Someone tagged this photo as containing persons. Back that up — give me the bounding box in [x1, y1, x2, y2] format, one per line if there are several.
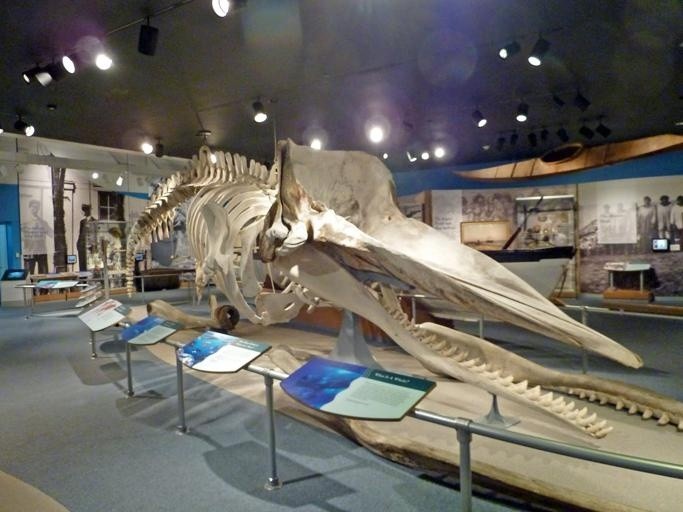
[600, 194, 683, 253]
[76, 202, 96, 273]
[21, 199, 54, 274]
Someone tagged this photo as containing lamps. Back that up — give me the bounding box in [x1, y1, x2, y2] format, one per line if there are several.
[252, 99, 268, 123]
[596, 124, 612, 138]
[509, 133, 519, 147]
[407, 150, 418, 162]
[495, 137, 505, 151]
[154, 142, 166, 159]
[515, 102, 529, 123]
[557, 128, 569, 142]
[498, 42, 521, 60]
[471, 110, 487, 128]
[552, 96, 566, 108]
[541, 129, 548, 140]
[11, 1, 246, 89]
[572, 95, 592, 112]
[527, 39, 553, 68]
[528, 133, 537, 147]
[16, 122, 35, 137]
[579, 126, 595, 139]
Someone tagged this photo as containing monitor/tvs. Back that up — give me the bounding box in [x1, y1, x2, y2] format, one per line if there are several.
[135, 253, 144, 262]
[651, 237, 669, 252]
[2, 268, 28, 281]
[66, 255, 77, 265]
